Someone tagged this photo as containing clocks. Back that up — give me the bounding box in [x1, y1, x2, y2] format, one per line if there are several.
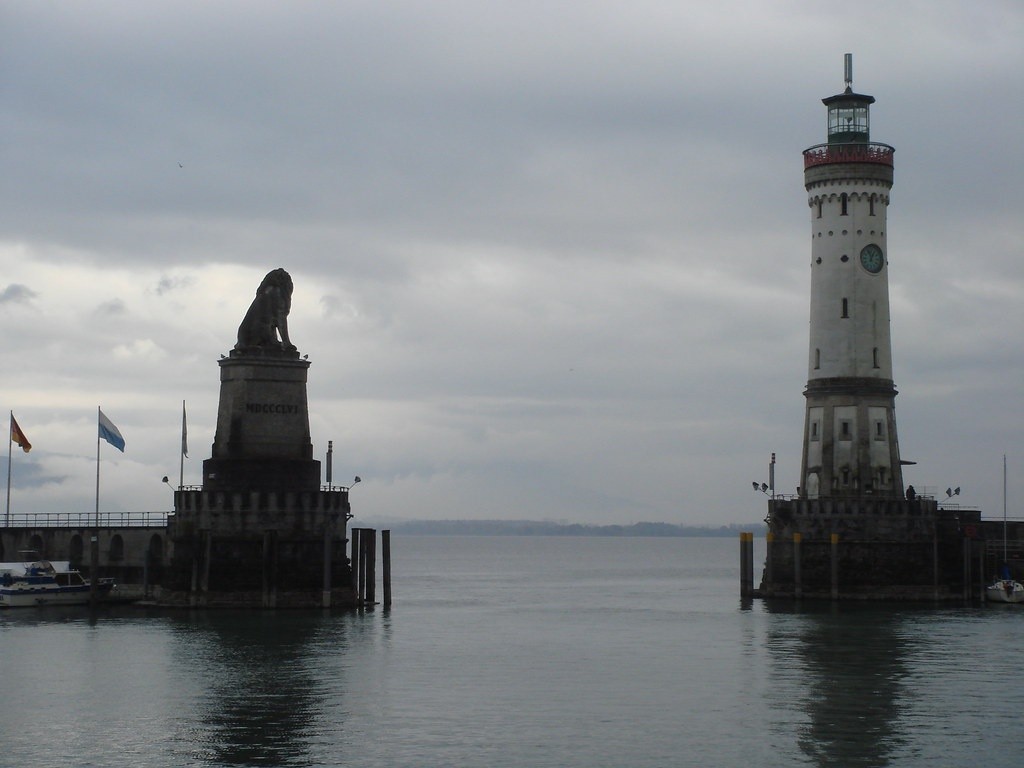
[861, 244, 883, 273]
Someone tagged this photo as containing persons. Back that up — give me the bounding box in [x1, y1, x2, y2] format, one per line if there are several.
[906, 485, 917, 500]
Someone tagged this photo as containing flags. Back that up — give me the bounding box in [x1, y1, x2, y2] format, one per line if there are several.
[182, 405, 189, 459]
[99, 411, 125, 454]
[11, 414, 32, 453]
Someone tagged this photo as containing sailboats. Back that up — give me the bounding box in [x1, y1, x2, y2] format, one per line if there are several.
[985, 453, 1024, 604]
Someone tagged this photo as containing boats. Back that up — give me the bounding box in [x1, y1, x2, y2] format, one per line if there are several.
[0, 559, 120, 607]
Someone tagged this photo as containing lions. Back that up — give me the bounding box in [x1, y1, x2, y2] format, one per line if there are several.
[235, 268, 298, 350]
